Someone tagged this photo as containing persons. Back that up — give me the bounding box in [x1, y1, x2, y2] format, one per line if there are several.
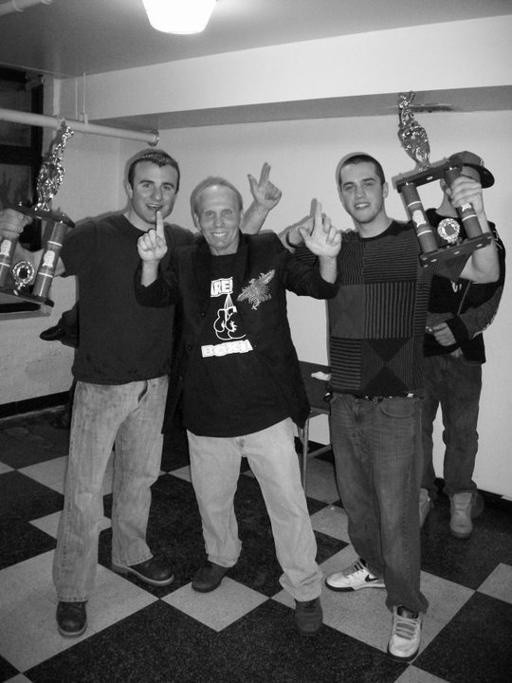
[137, 174, 346, 635]
[272, 149, 503, 662]
[0, 147, 282, 638]
[405, 150, 508, 540]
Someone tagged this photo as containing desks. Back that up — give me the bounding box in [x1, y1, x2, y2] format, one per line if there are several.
[298, 359, 331, 499]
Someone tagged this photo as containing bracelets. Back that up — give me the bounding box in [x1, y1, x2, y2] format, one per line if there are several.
[283, 227, 299, 251]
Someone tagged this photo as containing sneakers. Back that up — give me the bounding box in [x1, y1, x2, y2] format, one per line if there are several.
[420, 488, 433, 530]
[325, 559, 387, 592]
[386, 604, 423, 663]
[448, 491, 478, 540]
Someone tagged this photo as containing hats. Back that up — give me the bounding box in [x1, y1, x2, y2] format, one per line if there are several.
[446, 151, 495, 189]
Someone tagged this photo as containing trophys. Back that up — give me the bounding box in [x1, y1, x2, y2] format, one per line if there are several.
[389, 89, 496, 272]
[1, 115, 80, 308]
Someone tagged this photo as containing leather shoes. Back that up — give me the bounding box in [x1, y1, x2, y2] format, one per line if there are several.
[56, 599, 88, 637]
[109, 557, 175, 587]
[292, 597, 323, 638]
[192, 557, 229, 593]
